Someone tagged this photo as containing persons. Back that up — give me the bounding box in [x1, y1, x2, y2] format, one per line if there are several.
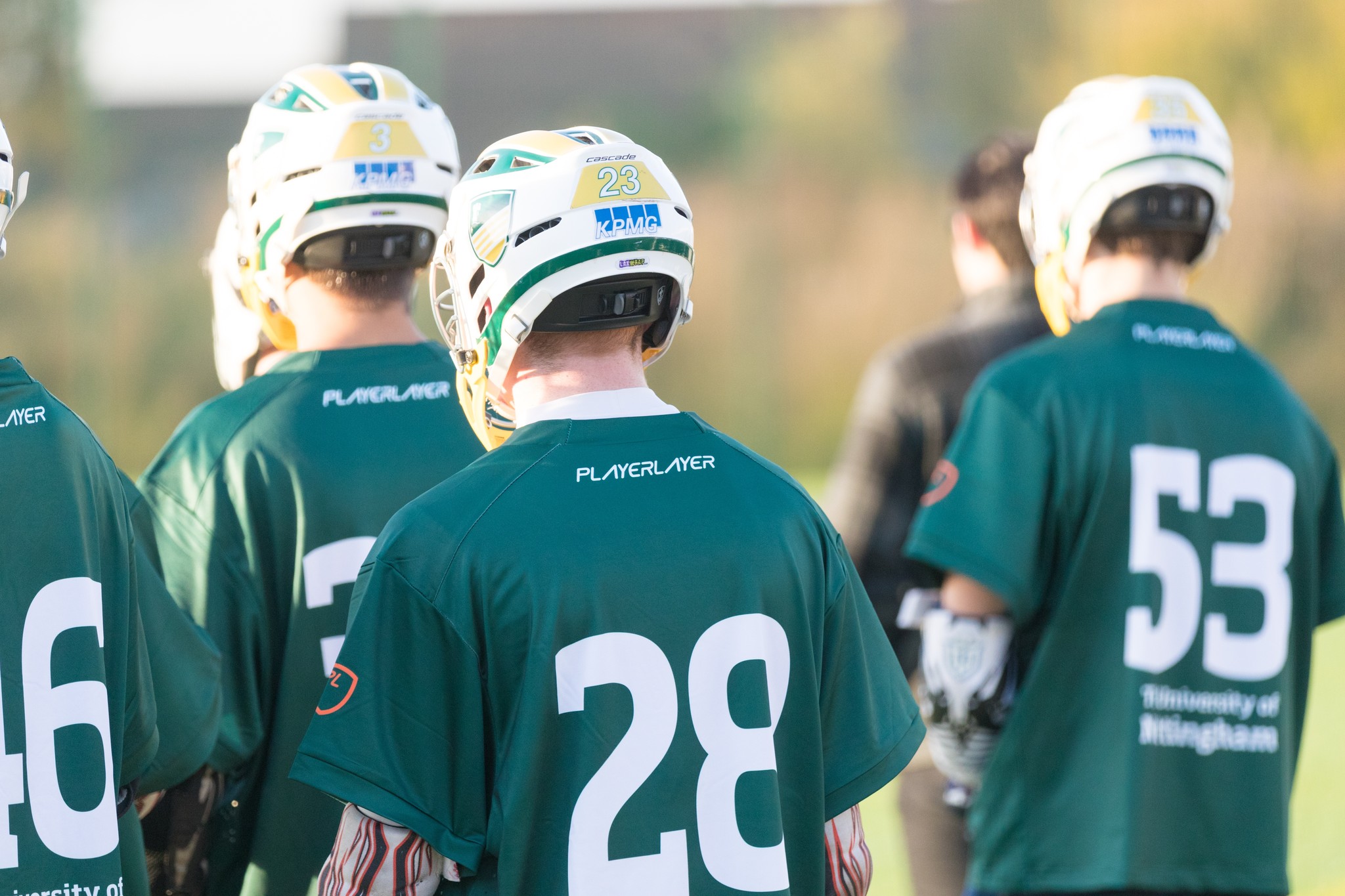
[818, 134, 1053, 896]
[905, 76, 1345, 896]
[0, 64, 927, 896]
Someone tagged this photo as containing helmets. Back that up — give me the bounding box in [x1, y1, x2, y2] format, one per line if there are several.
[1019, 73, 1236, 339]
[228, 61, 459, 349]
[429, 124, 696, 451]
[0, 119, 29, 259]
[210, 211, 272, 390]
